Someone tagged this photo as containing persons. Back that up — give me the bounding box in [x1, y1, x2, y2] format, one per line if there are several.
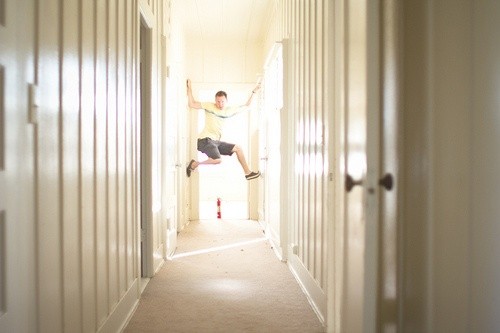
[183, 79, 262, 180]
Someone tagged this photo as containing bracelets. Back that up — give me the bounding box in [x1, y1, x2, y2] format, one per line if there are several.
[252, 90, 256, 93]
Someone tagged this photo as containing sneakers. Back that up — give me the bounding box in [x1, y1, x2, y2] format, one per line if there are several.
[185, 160, 195, 178]
[246, 172, 261, 180]
